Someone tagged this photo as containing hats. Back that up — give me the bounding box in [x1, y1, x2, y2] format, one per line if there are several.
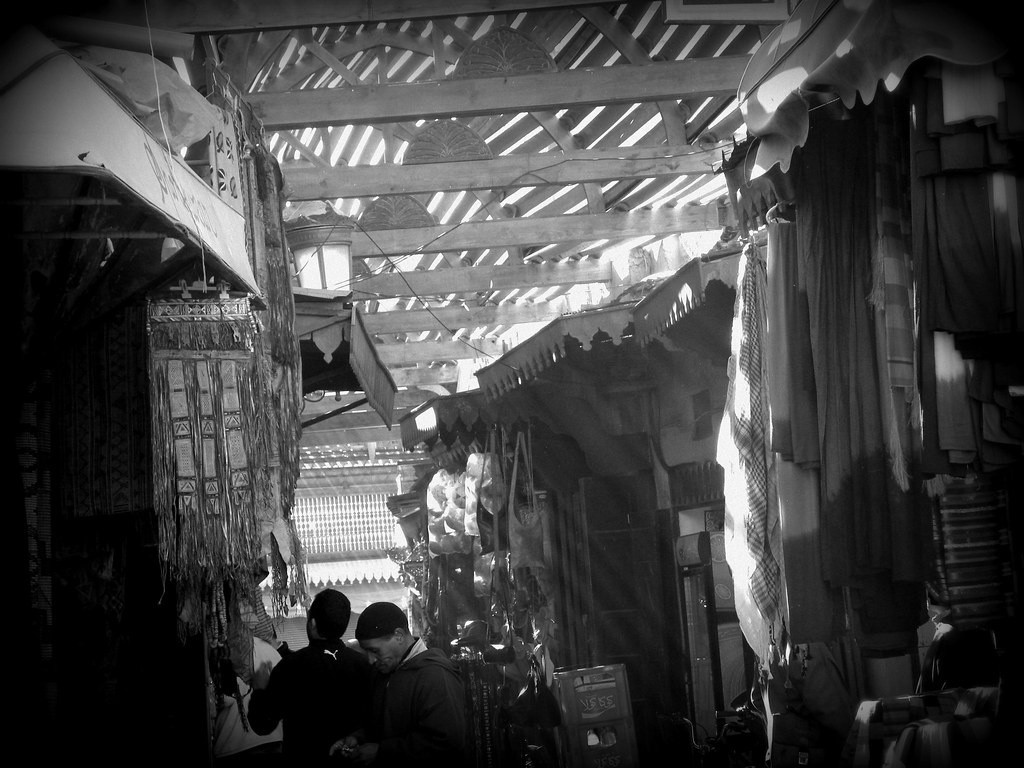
[356, 603, 408, 642]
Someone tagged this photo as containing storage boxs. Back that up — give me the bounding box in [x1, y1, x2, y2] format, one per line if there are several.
[553, 659, 638, 768]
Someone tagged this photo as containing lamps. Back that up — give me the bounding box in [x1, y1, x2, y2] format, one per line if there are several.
[286, 202, 357, 304]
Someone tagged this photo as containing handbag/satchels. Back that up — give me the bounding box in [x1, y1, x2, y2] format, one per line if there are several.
[478, 422, 559, 569]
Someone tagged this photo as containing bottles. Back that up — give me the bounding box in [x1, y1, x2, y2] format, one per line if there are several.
[587, 728, 617, 747]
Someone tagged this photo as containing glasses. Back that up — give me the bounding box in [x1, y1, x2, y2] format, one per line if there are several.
[307, 611, 312, 620]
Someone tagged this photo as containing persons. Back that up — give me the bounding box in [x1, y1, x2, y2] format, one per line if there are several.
[329, 602, 467, 768]
[211, 588, 376, 768]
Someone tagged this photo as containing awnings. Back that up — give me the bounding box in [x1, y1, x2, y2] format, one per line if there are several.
[740, 0, 1024, 181]
[294, 288, 398, 430]
[472, 301, 635, 403]
[1, 29, 265, 310]
[258, 557, 400, 592]
[632, 240, 764, 348]
[400, 390, 484, 449]
